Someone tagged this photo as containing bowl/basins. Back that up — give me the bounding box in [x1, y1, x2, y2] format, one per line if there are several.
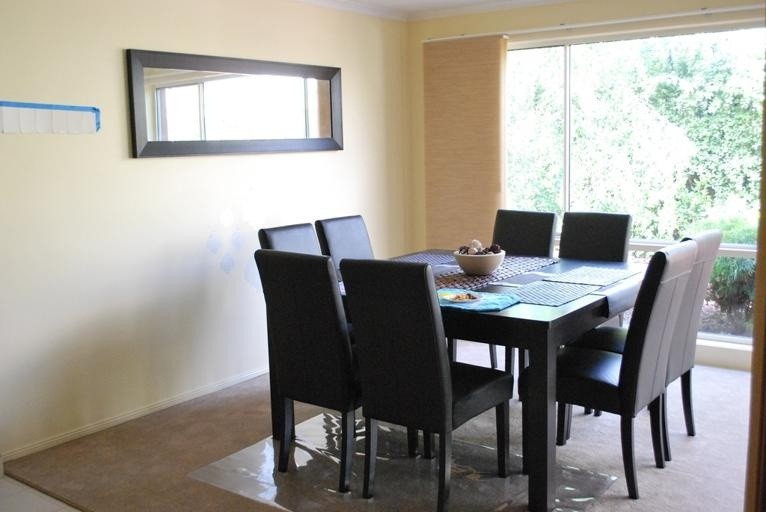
[453, 249, 506, 275]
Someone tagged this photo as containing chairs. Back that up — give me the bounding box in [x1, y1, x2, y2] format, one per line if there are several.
[314, 215, 374, 283]
[339, 258, 513, 511]
[517, 240, 697, 499]
[254, 249, 418, 493]
[258, 222, 322, 256]
[584, 232, 721, 461]
[447, 209, 555, 373]
[518, 212, 630, 416]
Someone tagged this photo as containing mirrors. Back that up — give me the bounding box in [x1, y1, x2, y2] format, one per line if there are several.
[126, 49, 342, 158]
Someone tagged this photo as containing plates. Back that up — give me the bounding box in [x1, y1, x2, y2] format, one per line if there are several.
[443, 293, 482, 303]
